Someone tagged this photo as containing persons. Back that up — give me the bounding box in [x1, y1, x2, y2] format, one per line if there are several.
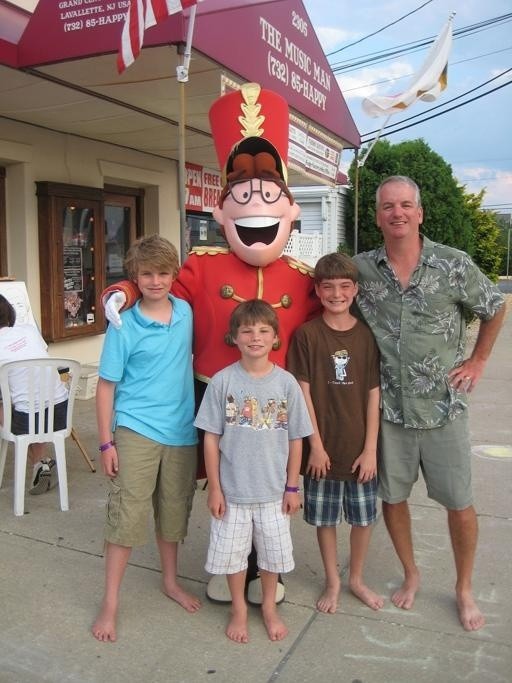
[0, 295, 71, 497]
[92, 237, 200, 641]
[97, 82, 329, 606]
[192, 299, 314, 644]
[337, 176, 505, 631]
[288, 253, 388, 616]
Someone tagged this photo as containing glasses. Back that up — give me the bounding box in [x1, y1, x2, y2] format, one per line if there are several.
[222, 177, 289, 204]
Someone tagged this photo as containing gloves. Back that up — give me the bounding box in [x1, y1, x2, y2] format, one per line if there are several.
[103, 290, 128, 330]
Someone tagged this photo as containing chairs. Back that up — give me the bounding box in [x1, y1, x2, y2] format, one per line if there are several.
[0, 357, 82, 517]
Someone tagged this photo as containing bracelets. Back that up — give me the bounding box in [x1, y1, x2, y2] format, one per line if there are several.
[97, 442, 113, 449]
[284, 486, 298, 492]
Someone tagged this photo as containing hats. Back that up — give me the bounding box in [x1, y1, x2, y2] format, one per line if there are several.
[205, 81, 289, 189]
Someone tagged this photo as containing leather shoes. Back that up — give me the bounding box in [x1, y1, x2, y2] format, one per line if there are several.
[245, 545, 287, 607]
[203, 556, 241, 609]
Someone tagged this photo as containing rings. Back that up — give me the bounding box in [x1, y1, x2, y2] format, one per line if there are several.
[464, 377, 471, 382]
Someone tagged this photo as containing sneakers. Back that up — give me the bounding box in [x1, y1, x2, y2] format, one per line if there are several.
[27, 459, 51, 496]
[48, 456, 59, 491]
